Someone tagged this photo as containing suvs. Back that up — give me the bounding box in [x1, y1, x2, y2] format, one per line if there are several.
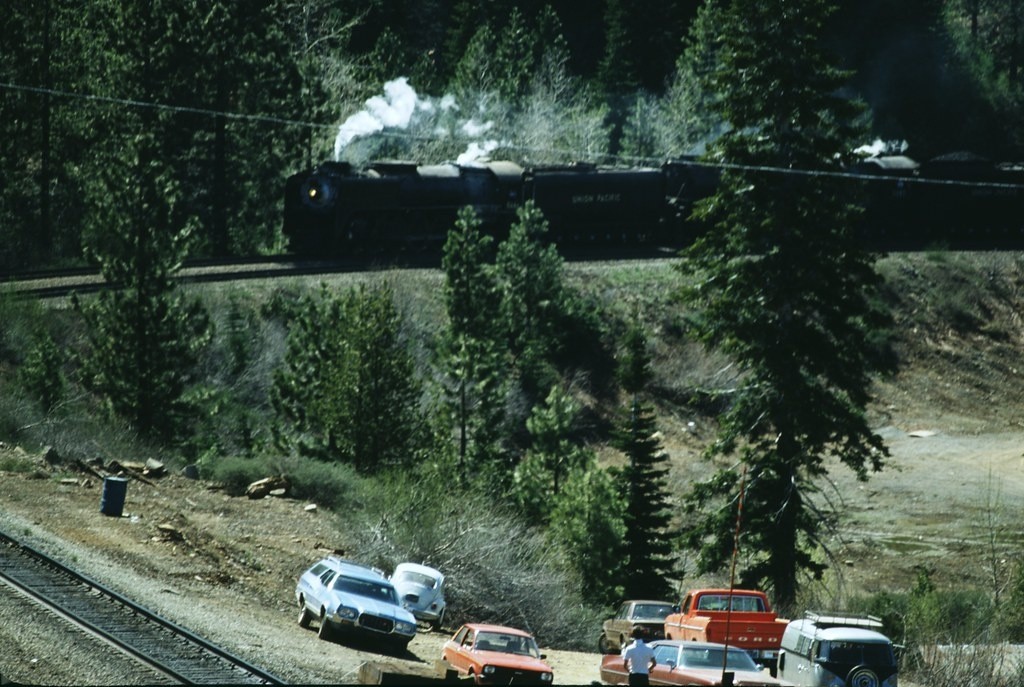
[294, 556, 416, 653]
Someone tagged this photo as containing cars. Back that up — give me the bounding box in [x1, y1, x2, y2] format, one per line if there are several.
[441, 625, 555, 686]
[601, 641, 793, 687]
[597, 600, 680, 655]
[382, 563, 448, 631]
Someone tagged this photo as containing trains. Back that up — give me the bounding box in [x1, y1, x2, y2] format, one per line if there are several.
[280, 153, 1024, 267]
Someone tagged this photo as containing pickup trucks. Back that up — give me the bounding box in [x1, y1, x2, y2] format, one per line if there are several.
[664, 590, 791, 673]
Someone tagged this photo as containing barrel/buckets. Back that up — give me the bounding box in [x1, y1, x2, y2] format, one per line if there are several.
[101, 477, 128, 517]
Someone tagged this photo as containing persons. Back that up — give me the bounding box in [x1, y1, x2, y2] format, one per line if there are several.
[623, 627, 656, 687]
